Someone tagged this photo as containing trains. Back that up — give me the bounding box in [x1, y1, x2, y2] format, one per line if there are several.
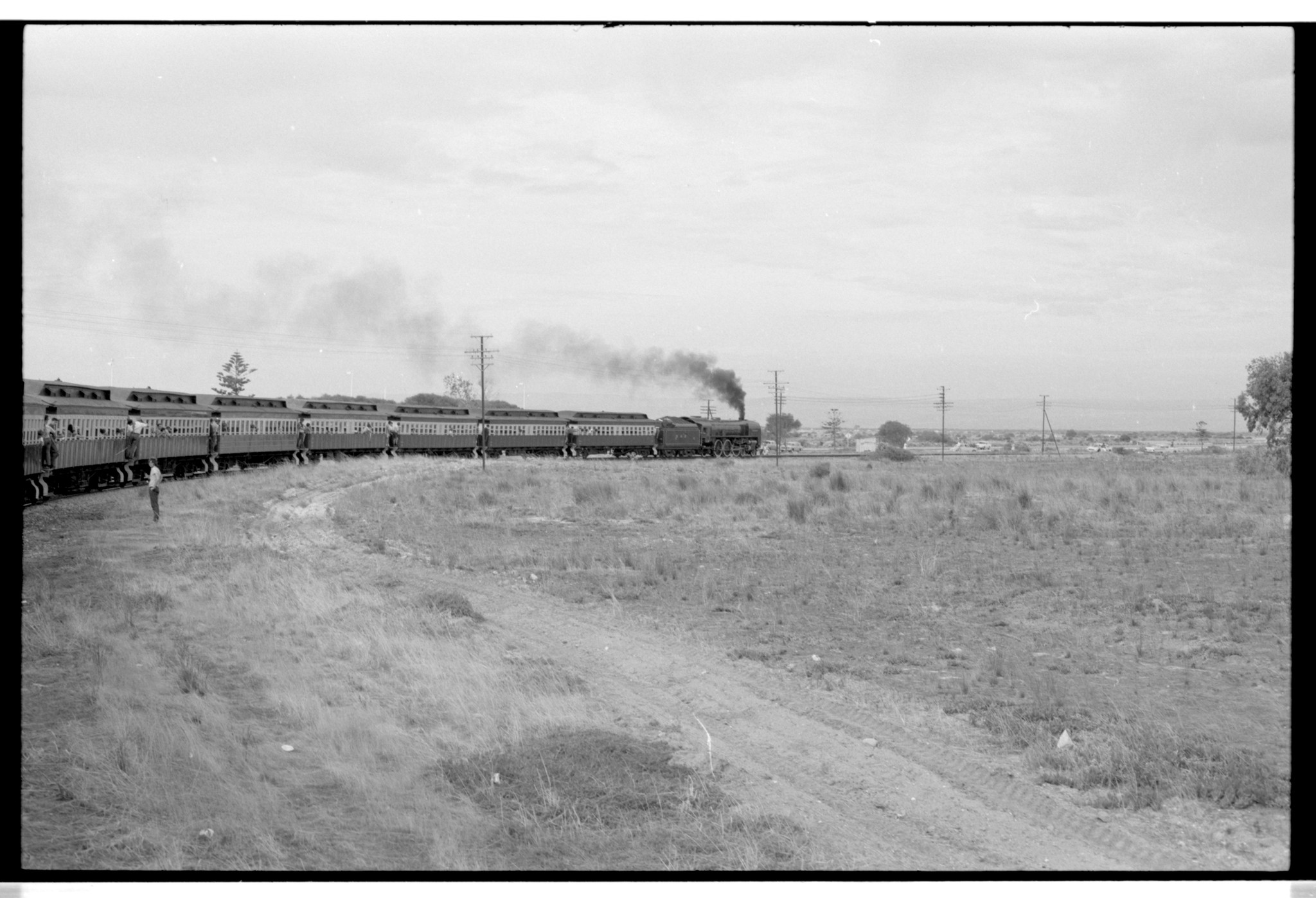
[22, 392, 761, 503]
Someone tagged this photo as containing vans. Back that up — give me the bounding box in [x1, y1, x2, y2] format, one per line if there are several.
[760, 440, 778, 451]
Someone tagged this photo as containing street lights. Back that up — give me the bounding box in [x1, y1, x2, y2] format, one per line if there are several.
[347, 371, 353, 396]
[519, 382, 525, 411]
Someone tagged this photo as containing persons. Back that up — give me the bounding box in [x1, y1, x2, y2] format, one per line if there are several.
[151, 423, 177, 438]
[297, 418, 310, 448]
[144, 457, 163, 523]
[481, 424, 489, 444]
[126, 417, 149, 460]
[580, 428, 598, 435]
[385, 420, 401, 447]
[449, 428, 457, 437]
[564, 423, 577, 447]
[759, 443, 769, 454]
[38, 415, 59, 469]
[62, 424, 124, 439]
[362, 424, 372, 434]
[411, 428, 415, 435]
[209, 418, 220, 456]
[222, 423, 227, 431]
[250, 425, 258, 433]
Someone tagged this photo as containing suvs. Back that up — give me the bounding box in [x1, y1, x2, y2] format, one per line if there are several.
[952, 443, 974, 453]
[779, 442, 803, 452]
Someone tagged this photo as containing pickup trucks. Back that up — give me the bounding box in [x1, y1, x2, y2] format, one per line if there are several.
[1145, 444, 1179, 454]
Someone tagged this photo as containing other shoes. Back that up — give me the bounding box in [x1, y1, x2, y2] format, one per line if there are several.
[126, 459, 138, 466]
[41, 464, 54, 473]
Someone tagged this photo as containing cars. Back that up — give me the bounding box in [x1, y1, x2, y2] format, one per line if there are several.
[1086, 443, 1111, 453]
[976, 441, 991, 450]
